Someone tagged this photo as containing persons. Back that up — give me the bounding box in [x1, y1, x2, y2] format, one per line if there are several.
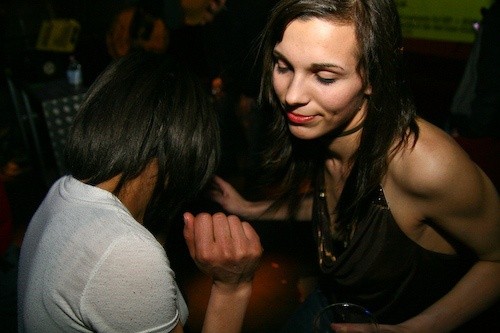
[208, 0, 500, 333]
[16, 48, 263, 333]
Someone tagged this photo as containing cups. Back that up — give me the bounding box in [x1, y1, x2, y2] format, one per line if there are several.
[313, 302, 377, 333]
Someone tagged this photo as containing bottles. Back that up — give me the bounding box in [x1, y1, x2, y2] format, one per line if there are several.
[64, 57, 82, 90]
